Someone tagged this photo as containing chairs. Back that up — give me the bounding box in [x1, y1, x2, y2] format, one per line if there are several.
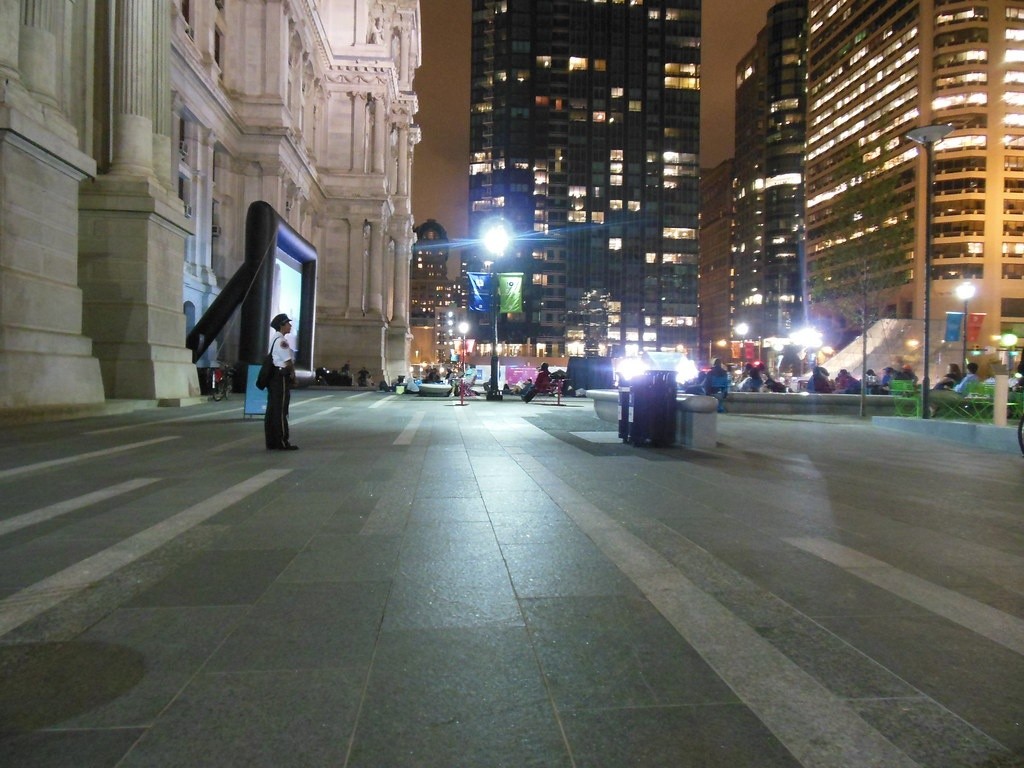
[889, 380, 920, 418]
[966, 383, 993, 424]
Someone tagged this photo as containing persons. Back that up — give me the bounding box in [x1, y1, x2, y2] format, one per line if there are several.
[700, 359, 983, 413]
[256, 314, 299, 451]
[316, 361, 535, 398]
[521, 362, 551, 403]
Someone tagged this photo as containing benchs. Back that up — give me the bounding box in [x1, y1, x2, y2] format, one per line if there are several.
[585, 388, 720, 448]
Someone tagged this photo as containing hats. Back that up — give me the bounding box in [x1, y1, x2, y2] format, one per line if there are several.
[883, 367, 893, 372]
[902, 364, 911, 370]
[271, 313, 292, 330]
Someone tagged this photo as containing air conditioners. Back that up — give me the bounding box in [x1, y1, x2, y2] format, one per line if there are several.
[184, 205, 193, 217]
[217, 0, 226, 10]
[211, 225, 222, 236]
[179, 140, 190, 156]
[286, 201, 292, 211]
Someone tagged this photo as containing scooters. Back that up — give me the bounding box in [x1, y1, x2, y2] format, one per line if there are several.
[707, 388, 727, 413]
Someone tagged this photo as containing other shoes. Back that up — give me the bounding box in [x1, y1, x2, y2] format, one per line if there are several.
[277, 445, 297, 451]
[521, 394, 527, 402]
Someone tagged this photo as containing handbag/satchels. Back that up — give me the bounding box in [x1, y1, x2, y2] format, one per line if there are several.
[256, 337, 282, 390]
[550, 369, 568, 379]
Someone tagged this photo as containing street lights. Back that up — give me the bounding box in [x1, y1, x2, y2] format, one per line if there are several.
[1000, 336, 1018, 379]
[956, 281, 975, 381]
[478, 223, 510, 401]
[737, 322, 749, 373]
[457, 321, 470, 405]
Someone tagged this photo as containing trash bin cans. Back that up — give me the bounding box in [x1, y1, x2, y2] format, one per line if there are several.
[616, 368, 678, 447]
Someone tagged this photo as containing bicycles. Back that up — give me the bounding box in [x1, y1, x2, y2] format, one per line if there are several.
[210, 363, 237, 402]
